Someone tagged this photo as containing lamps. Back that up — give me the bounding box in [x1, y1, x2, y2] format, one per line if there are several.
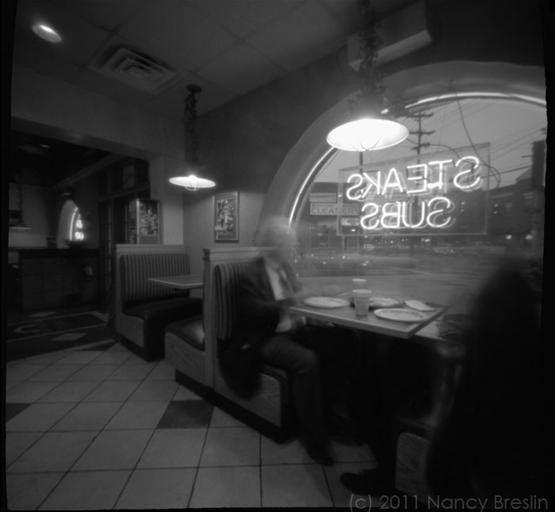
[165, 82, 217, 191]
[8, 174, 32, 230]
[325, 0, 410, 154]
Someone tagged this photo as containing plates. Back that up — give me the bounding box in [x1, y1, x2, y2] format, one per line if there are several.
[303, 293, 426, 325]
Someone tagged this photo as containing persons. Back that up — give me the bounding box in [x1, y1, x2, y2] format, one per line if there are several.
[237, 215, 338, 466]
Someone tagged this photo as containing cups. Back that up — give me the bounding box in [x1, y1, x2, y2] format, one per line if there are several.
[350, 277, 373, 318]
